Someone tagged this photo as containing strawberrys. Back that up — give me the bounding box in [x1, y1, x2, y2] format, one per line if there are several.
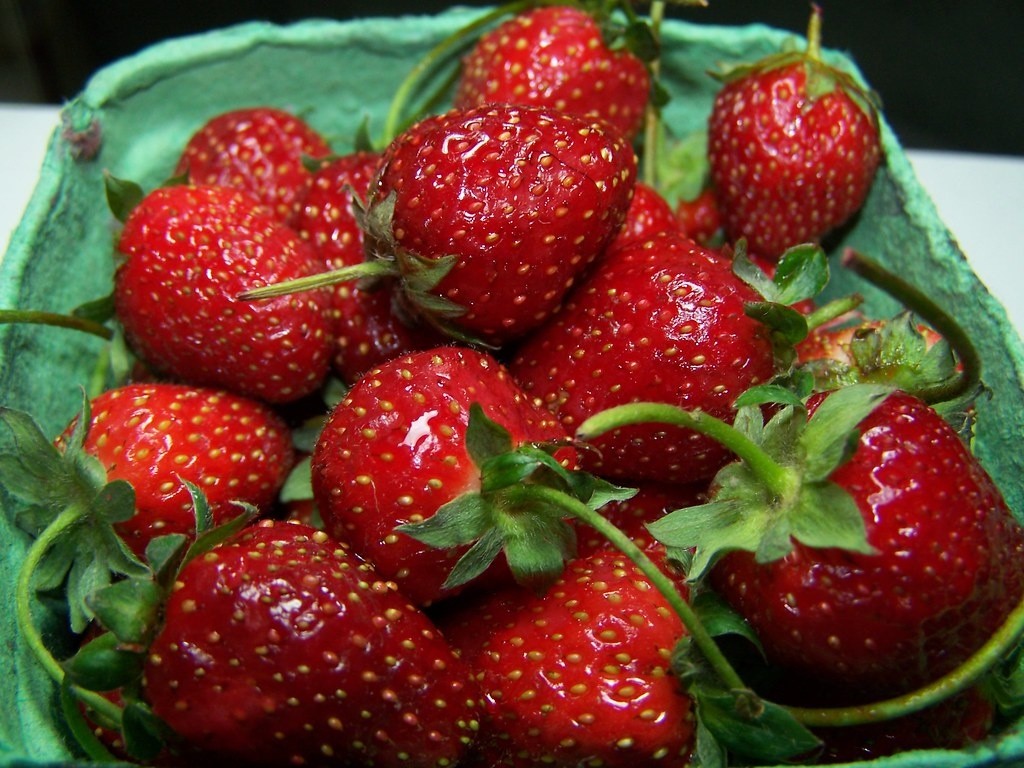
[2, 0, 1024, 768]
